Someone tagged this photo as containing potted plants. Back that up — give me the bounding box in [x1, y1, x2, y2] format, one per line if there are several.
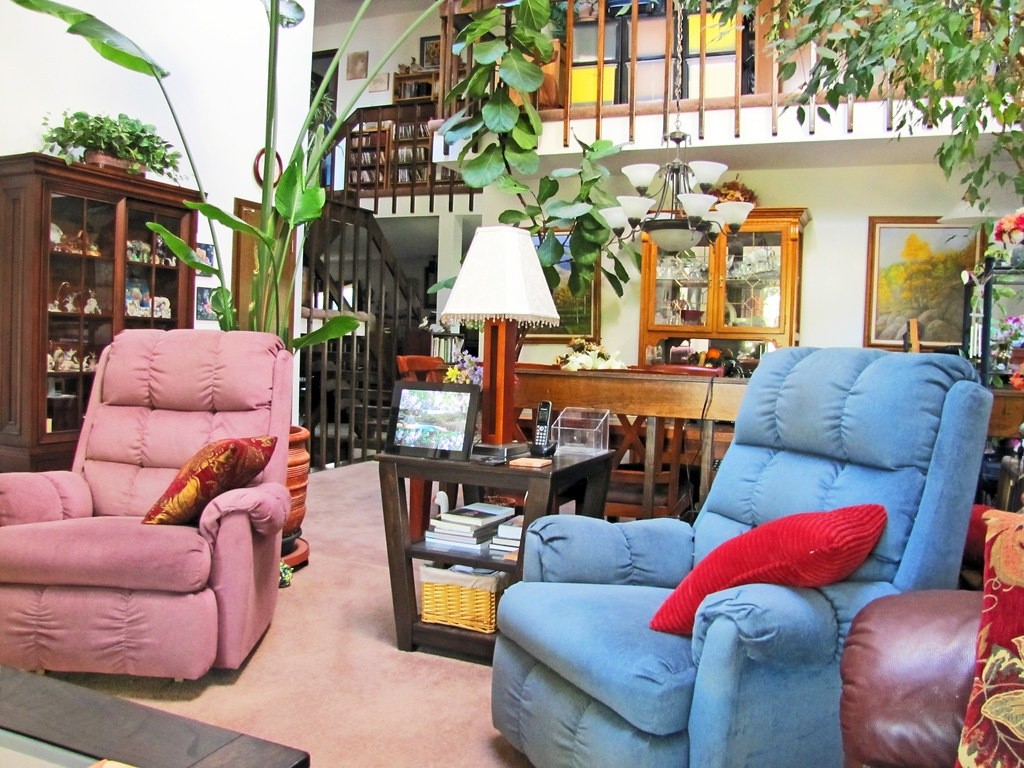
[8, 0, 451, 582]
[442, 1, 644, 445]
[34, 106, 185, 189]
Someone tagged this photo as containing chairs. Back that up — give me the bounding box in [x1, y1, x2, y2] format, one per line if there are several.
[394, 353, 460, 541]
[606, 364, 724, 518]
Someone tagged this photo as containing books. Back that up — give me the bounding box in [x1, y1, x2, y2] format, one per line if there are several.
[350, 151, 385, 165]
[397, 167, 429, 182]
[424, 501, 516, 549]
[397, 81, 432, 99]
[351, 136, 372, 147]
[488, 515, 524, 552]
[392, 147, 429, 163]
[350, 169, 385, 183]
[352, 120, 394, 130]
[398, 123, 432, 139]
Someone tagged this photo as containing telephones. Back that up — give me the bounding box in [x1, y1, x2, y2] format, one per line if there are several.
[529, 400, 557, 457]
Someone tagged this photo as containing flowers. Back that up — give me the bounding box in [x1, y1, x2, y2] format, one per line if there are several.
[969, 205, 1024, 394]
[551, 334, 626, 371]
[706, 172, 759, 208]
[1007, 427, 1024, 471]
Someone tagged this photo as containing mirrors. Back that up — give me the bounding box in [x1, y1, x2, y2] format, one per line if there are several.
[254, 148, 283, 190]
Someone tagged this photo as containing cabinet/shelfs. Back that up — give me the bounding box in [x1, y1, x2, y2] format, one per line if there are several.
[0, 152, 208, 474]
[344, 70, 484, 197]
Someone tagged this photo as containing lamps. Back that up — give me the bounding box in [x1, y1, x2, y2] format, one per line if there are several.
[598, 0, 754, 282]
[435, 225, 562, 462]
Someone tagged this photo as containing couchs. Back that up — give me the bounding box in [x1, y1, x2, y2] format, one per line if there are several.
[491, 347, 993, 767]
[839, 502, 1024, 768]
[0, 329, 293, 681]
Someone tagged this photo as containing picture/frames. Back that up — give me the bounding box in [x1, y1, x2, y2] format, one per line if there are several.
[419, 35, 442, 69]
[859, 216, 983, 357]
[515, 223, 602, 346]
[344, 50, 368, 80]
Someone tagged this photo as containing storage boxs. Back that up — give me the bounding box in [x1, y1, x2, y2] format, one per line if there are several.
[551, 405, 609, 457]
[416, 563, 509, 636]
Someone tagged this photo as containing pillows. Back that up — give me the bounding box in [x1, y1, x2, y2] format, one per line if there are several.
[647, 501, 889, 634]
[954, 506, 1024, 768]
[140, 435, 280, 523]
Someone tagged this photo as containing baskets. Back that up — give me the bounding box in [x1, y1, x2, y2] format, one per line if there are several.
[419, 560, 515, 634]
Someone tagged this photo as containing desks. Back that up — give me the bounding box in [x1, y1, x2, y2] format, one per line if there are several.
[511, 366, 750, 510]
[365, 441, 616, 661]
[0, 654, 310, 766]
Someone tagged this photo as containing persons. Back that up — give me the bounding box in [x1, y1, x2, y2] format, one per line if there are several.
[460, 509, 483, 517]
